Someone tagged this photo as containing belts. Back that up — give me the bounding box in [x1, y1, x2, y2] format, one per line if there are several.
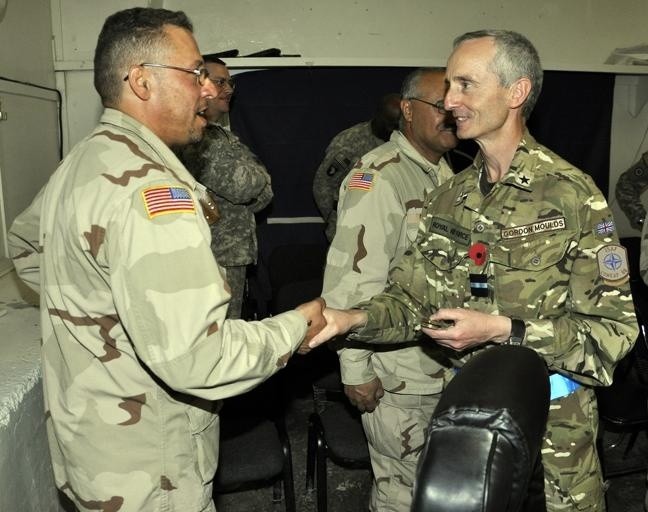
[548, 373, 581, 403]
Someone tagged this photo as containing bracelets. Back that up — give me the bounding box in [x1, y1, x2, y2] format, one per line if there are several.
[637, 215, 645, 224]
[499, 315, 527, 347]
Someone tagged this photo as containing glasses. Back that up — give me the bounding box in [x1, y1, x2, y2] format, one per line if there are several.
[121, 61, 209, 86]
[210, 78, 236, 90]
[407, 96, 450, 115]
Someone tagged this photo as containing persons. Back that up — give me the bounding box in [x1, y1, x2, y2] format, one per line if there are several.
[319, 69, 462, 512]
[8, 8, 329, 512]
[616, 148, 648, 232]
[312, 87, 400, 241]
[639, 208, 648, 286]
[310, 28, 638, 509]
[182, 58, 276, 318]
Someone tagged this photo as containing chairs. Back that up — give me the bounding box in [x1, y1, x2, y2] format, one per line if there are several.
[206, 266, 295, 507]
[275, 344, 373, 508]
[595, 367, 648, 480]
[406, 343, 553, 512]
